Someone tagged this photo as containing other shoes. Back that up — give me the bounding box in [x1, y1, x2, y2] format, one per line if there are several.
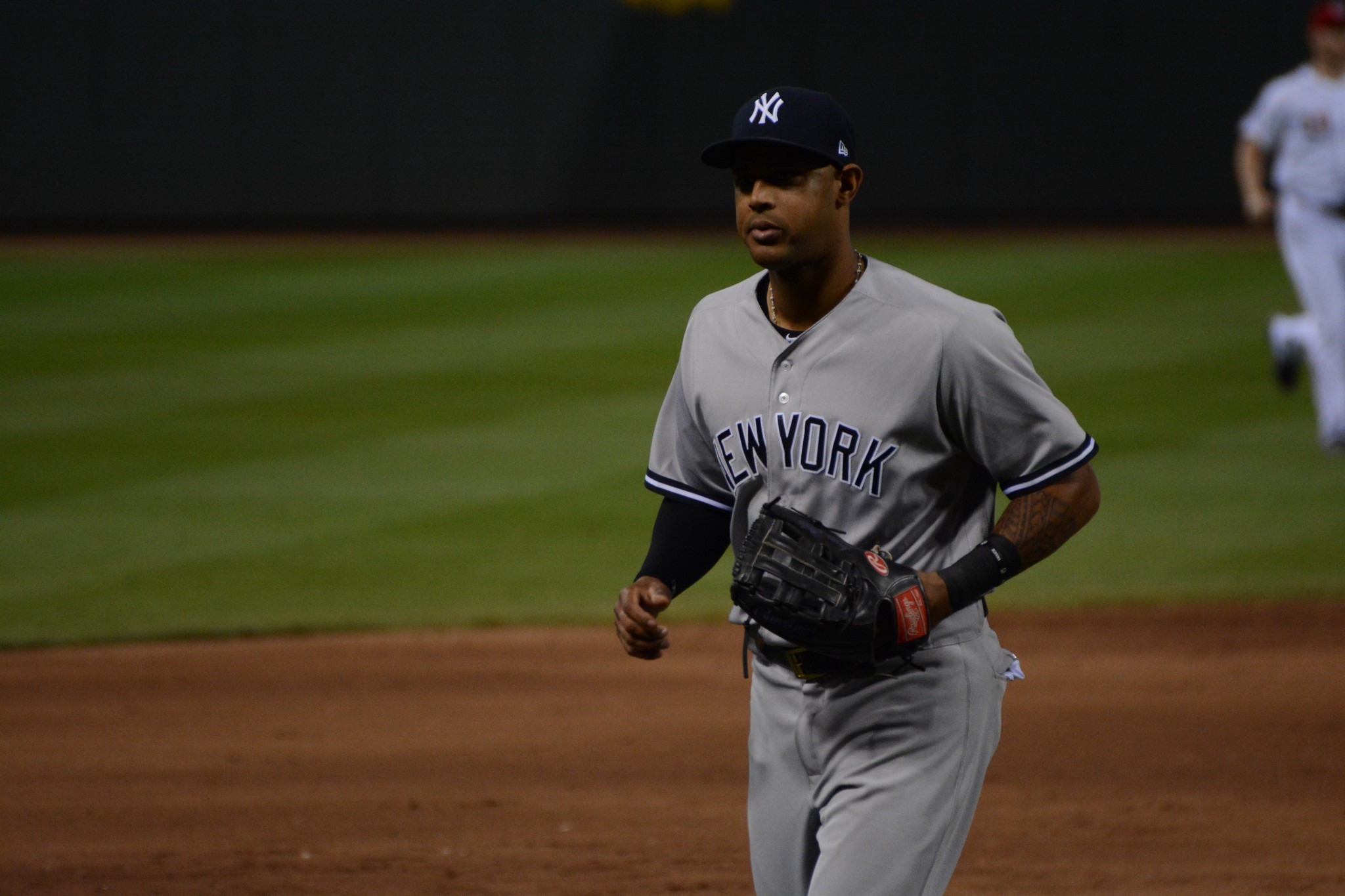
[1275, 356, 1299, 393]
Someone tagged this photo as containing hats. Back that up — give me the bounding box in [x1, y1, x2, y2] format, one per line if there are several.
[1307, 1, 1345, 29]
[700, 87, 856, 166]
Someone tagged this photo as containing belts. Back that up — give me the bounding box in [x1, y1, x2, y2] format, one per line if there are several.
[760, 595, 989, 680]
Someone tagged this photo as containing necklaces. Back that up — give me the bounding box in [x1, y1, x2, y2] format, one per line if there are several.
[771, 248, 863, 328]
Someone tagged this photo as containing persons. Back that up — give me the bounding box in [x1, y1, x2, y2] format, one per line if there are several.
[1236, 0, 1345, 447]
[615, 86, 1101, 896]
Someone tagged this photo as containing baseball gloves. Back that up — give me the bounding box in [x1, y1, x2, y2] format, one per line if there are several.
[732, 507, 928, 677]
